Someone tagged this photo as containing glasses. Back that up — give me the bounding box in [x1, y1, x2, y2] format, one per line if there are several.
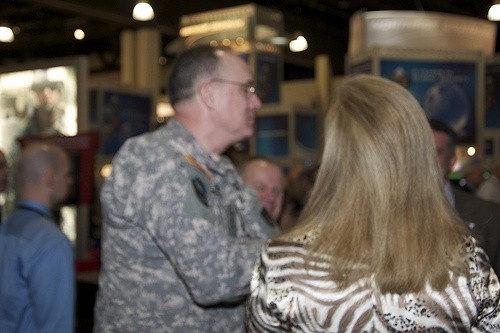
[210, 78, 254, 97]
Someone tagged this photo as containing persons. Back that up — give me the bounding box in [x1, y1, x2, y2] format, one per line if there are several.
[235, 156, 286, 221]
[426, 123, 500, 282]
[246, 73, 499, 332]
[92, 44, 283, 333]
[13, 68, 67, 230]
[288, 162, 317, 209]
[1, 138, 78, 333]
